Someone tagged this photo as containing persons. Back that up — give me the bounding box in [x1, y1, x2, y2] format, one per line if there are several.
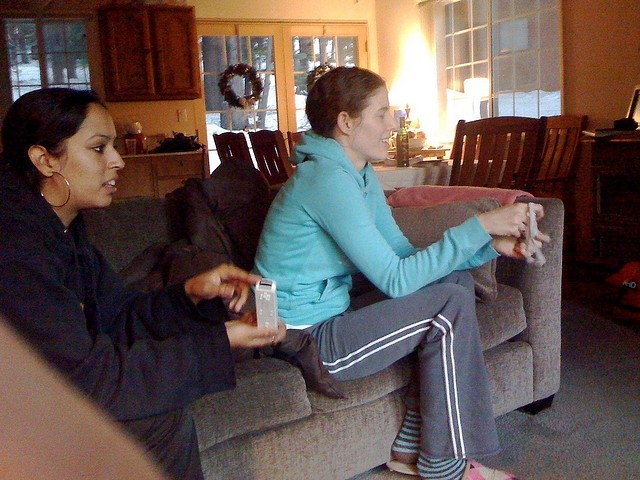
[249, 67, 550, 479]
[0, 88, 285, 479]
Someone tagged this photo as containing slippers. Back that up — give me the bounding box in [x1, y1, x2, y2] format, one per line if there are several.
[461, 458, 516, 480]
[385, 458, 418, 477]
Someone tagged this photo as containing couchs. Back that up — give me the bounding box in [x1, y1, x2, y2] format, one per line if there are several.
[84, 172, 566, 477]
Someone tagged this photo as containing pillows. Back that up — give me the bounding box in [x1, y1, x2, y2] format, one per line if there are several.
[389, 197, 500, 301]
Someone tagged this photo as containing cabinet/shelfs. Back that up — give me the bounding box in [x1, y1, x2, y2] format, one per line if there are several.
[579, 136, 640, 269]
[107, 154, 156, 197]
[104, 2, 202, 101]
[153, 149, 209, 198]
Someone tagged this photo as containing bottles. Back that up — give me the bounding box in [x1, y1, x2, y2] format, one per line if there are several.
[394, 116, 413, 169]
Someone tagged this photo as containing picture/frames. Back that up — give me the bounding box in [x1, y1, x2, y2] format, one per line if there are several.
[627, 86, 640, 131]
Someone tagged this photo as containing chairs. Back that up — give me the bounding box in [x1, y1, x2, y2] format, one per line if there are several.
[248, 130, 294, 187]
[448, 114, 538, 191]
[535, 115, 586, 195]
[214, 132, 248, 171]
[288, 129, 309, 148]
